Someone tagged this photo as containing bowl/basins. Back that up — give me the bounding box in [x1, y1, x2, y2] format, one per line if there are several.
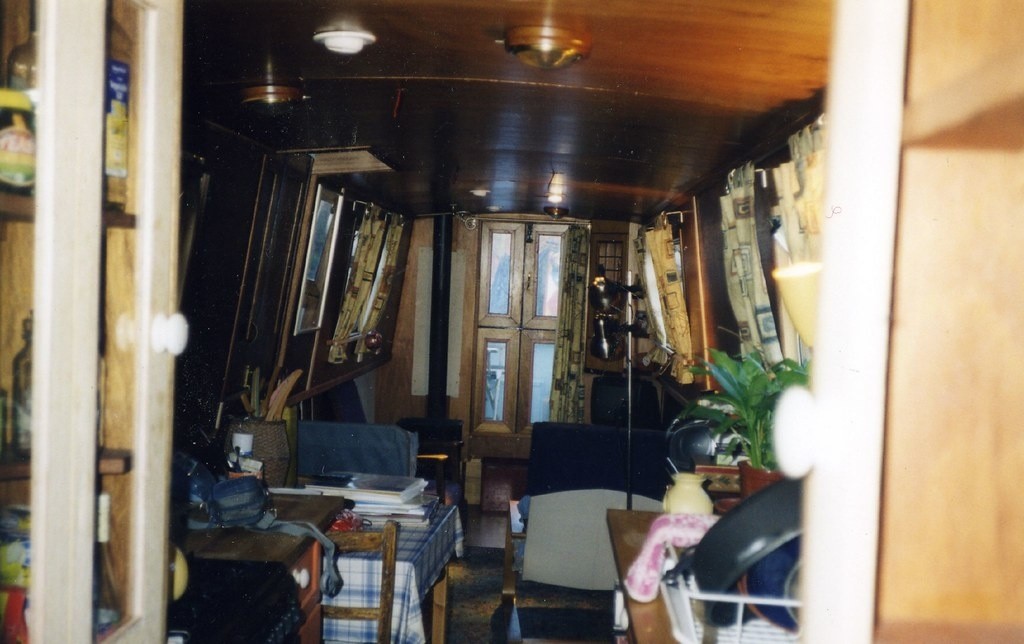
[694, 477, 802, 627]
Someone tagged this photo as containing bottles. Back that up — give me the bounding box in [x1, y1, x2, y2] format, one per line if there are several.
[11, 318, 33, 458]
[7, 0, 38, 113]
[662, 472, 713, 517]
[94, 493, 122, 634]
[105, 26, 129, 210]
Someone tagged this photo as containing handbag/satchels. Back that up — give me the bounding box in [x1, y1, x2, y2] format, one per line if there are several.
[211, 477, 266, 526]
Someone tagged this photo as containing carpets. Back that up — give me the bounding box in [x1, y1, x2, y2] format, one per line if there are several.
[423, 544, 615, 643]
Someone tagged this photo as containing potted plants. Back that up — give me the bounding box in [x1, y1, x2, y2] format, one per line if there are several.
[676, 346, 808, 503]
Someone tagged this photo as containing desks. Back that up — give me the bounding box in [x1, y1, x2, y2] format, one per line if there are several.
[607, 509, 715, 644]
[298, 482, 464, 644]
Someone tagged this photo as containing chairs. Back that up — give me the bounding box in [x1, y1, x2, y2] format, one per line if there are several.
[298, 421, 419, 478]
[320, 520, 400, 643]
[312, 380, 468, 528]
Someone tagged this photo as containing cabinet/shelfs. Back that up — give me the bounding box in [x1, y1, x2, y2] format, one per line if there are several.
[0, 1, 182, 644]
[169, 494, 345, 644]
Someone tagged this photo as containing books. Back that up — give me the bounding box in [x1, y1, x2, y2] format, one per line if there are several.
[305, 471, 439, 527]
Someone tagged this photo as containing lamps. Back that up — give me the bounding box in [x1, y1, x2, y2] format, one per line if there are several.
[590, 264, 650, 359]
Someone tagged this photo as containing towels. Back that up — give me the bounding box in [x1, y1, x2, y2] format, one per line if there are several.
[621, 511, 723, 604]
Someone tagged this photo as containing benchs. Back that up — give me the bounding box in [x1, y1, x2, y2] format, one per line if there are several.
[501, 421, 707, 643]
